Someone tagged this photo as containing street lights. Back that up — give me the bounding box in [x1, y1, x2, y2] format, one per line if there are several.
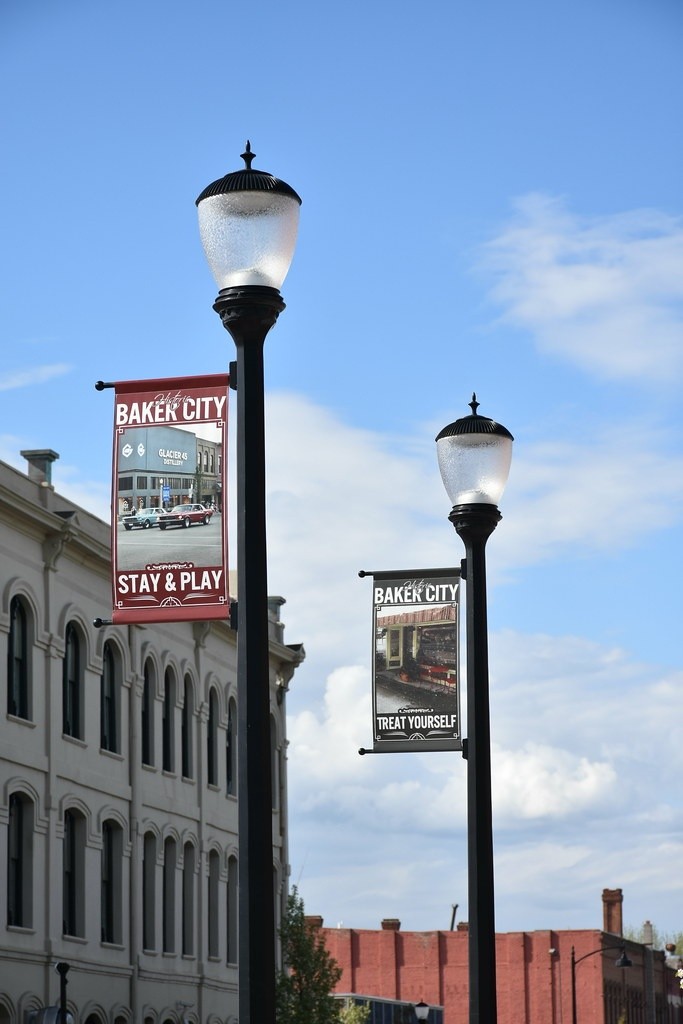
[430, 394, 515, 1024]
[193, 138, 303, 1024]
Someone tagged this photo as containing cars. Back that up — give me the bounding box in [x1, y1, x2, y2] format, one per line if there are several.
[155, 503, 212, 530]
[121, 507, 167, 531]
